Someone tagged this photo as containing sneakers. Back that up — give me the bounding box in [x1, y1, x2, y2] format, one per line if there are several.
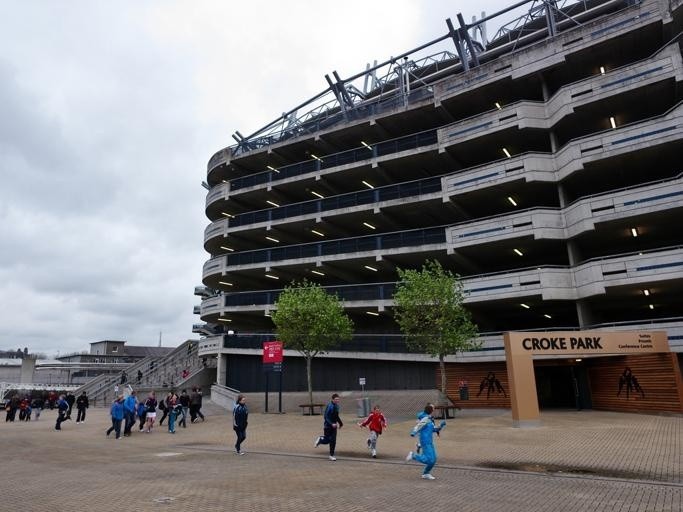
[329, 456, 336, 460]
[422, 474, 435, 479]
[368, 438, 376, 458]
[406, 443, 421, 461]
[314, 436, 323, 448]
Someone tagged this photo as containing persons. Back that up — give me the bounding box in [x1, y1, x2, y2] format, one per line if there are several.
[104, 385, 207, 441]
[357, 404, 387, 460]
[414, 404, 436, 456]
[110, 340, 210, 390]
[403, 403, 445, 482]
[231, 394, 250, 456]
[313, 393, 344, 461]
[0, 386, 88, 434]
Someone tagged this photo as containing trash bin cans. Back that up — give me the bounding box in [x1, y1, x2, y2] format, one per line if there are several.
[355, 398, 371, 418]
